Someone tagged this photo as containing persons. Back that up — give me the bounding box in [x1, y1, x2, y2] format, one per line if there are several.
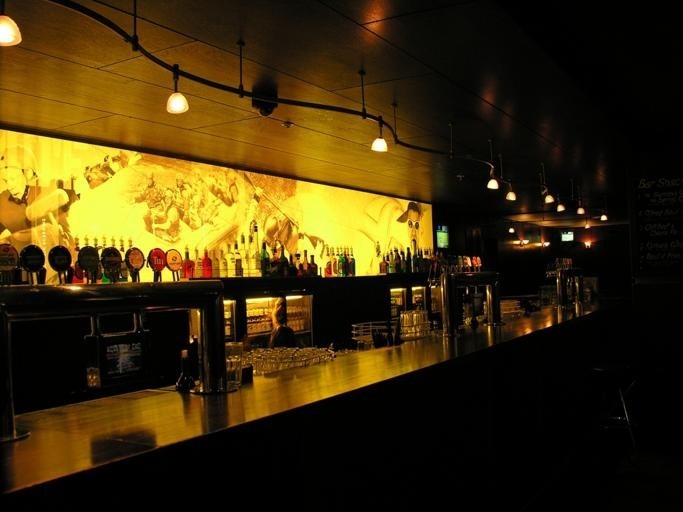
[0, 146, 423, 285]
[267, 297, 306, 348]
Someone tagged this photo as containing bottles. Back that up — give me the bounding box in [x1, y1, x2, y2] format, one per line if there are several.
[542, 257, 592, 307]
[388, 294, 438, 316]
[173, 349, 194, 392]
[71, 225, 316, 283]
[323, 244, 356, 277]
[222, 303, 308, 334]
[378, 246, 444, 276]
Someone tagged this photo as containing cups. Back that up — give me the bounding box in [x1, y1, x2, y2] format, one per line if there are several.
[399, 309, 429, 340]
[223, 345, 333, 390]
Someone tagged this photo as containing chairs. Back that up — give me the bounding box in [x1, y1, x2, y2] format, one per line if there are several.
[351, 320, 388, 353]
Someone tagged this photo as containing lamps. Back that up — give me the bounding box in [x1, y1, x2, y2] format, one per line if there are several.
[0, 2, 26, 52]
[475, 159, 610, 225]
[163, 58, 191, 117]
[368, 111, 390, 155]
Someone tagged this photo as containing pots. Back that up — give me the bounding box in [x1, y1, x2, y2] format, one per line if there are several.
[0, 242, 183, 284]
